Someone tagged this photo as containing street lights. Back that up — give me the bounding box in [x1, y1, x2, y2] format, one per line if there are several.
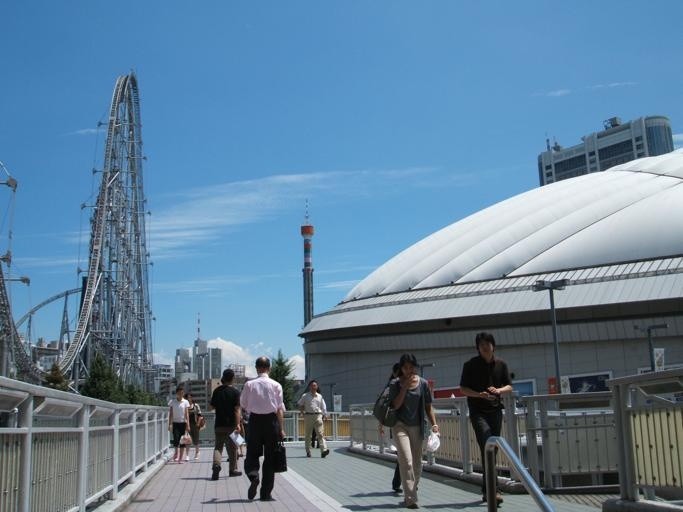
[531, 277, 571, 393]
[417, 362, 435, 379]
[646, 323, 669, 372]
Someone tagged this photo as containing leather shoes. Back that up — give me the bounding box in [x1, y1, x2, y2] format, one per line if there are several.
[496, 492, 504, 503]
[321, 449, 329, 458]
[213, 465, 219, 480]
[230, 471, 242, 477]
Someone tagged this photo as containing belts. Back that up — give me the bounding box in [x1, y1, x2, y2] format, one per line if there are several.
[306, 412, 322, 414]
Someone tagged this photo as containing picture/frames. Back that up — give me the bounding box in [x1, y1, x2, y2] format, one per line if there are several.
[637, 363, 681, 377]
[567, 369, 613, 393]
[499, 376, 540, 412]
[430, 385, 468, 399]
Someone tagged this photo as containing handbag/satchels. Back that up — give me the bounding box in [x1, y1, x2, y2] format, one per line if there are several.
[372, 387, 396, 427]
[275, 441, 287, 473]
[180, 433, 192, 445]
[198, 417, 206, 430]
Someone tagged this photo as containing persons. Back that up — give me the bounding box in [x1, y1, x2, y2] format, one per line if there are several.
[313, 387, 326, 448]
[165, 386, 191, 466]
[184, 394, 206, 460]
[378, 364, 417, 492]
[241, 355, 287, 502]
[204, 368, 242, 480]
[458, 329, 512, 505]
[389, 352, 441, 508]
[293, 379, 330, 458]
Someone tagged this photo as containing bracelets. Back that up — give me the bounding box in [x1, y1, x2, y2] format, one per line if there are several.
[431, 424, 438, 428]
